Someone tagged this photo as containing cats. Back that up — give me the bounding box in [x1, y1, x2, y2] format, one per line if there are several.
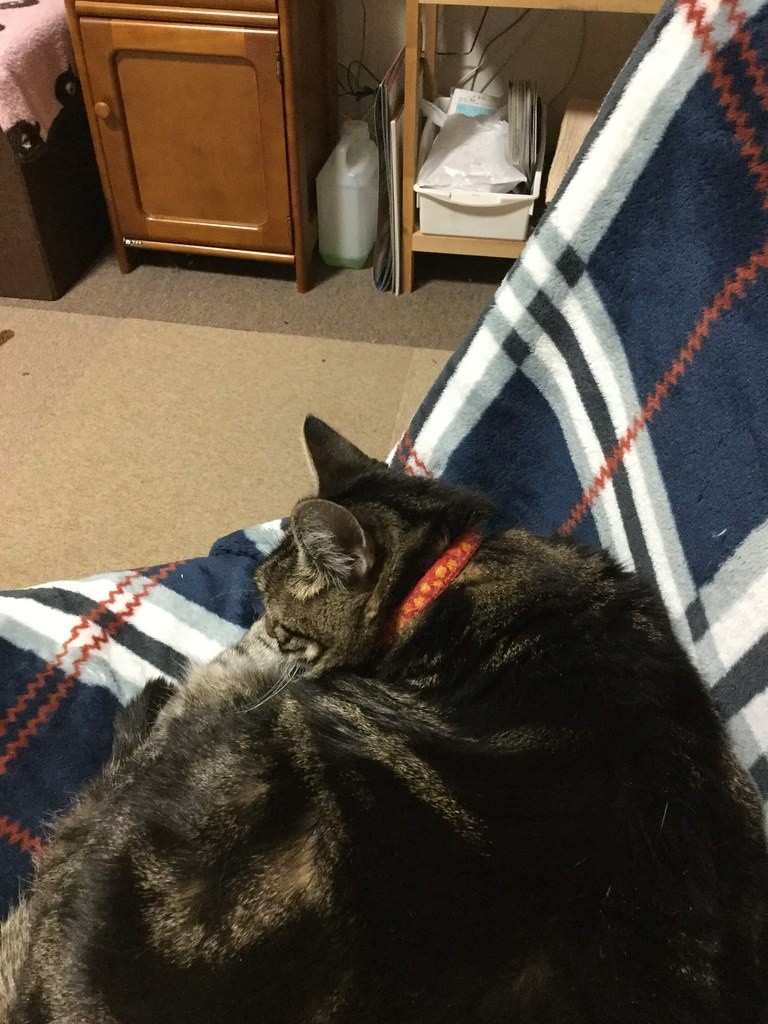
[0, 413, 768, 1024]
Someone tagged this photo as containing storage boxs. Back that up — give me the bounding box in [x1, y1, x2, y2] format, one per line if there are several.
[414, 96, 547, 241]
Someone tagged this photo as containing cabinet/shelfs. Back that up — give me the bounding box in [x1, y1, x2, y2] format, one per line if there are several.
[397, 0, 664, 295]
[64, 0, 344, 296]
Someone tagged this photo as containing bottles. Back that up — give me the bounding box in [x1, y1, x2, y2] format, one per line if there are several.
[316, 119, 381, 271]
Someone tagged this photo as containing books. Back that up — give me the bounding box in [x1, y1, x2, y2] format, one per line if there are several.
[370, 40, 428, 300]
[445, 75, 537, 195]
